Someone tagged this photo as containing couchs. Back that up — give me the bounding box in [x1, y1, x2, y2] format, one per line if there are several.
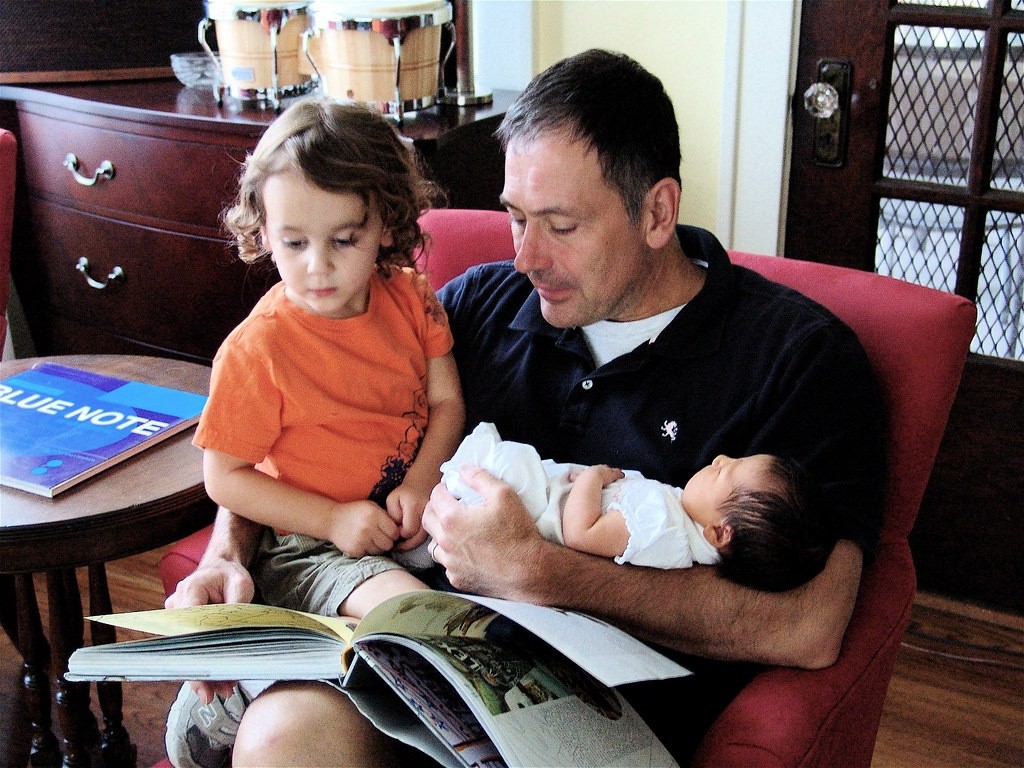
[384, 208, 978, 768]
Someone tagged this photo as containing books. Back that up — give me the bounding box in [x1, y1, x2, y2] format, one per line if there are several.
[1, 363, 210, 499]
[63, 591, 696, 768]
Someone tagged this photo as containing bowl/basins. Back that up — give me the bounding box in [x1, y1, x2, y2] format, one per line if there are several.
[170, 51, 224, 90]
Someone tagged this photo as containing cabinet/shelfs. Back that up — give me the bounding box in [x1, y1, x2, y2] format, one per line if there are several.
[0, 77, 519, 368]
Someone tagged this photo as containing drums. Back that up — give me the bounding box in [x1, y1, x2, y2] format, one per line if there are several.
[306, 0, 452, 113]
[197, 0, 318, 102]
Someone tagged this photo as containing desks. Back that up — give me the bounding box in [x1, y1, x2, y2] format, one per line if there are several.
[0, 354, 218, 768]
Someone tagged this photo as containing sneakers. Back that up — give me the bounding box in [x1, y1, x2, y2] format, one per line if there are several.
[165, 680, 275, 768]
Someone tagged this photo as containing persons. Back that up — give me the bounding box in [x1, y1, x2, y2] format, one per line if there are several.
[192, 97, 465, 619]
[165, 47, 897, 768]
[441, 421, 838, 595]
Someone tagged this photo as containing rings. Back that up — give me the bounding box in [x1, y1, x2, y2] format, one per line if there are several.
[432, 543, 439, 563]
